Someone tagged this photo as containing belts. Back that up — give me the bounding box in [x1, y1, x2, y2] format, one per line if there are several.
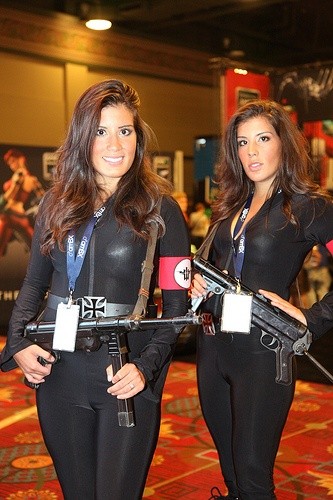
[47, 292, 158, 321]
[201, 313, 256, 345]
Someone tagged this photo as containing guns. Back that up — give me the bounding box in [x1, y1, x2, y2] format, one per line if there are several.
[189, 255, 333, 386]
[22, 314, 203, 427]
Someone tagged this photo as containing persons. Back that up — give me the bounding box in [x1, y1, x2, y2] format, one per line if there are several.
[0, 148, 45, 256]
[0, 79, 192, 499]
[188, 203, 209, 249]
[187, 99, 332, 500]
